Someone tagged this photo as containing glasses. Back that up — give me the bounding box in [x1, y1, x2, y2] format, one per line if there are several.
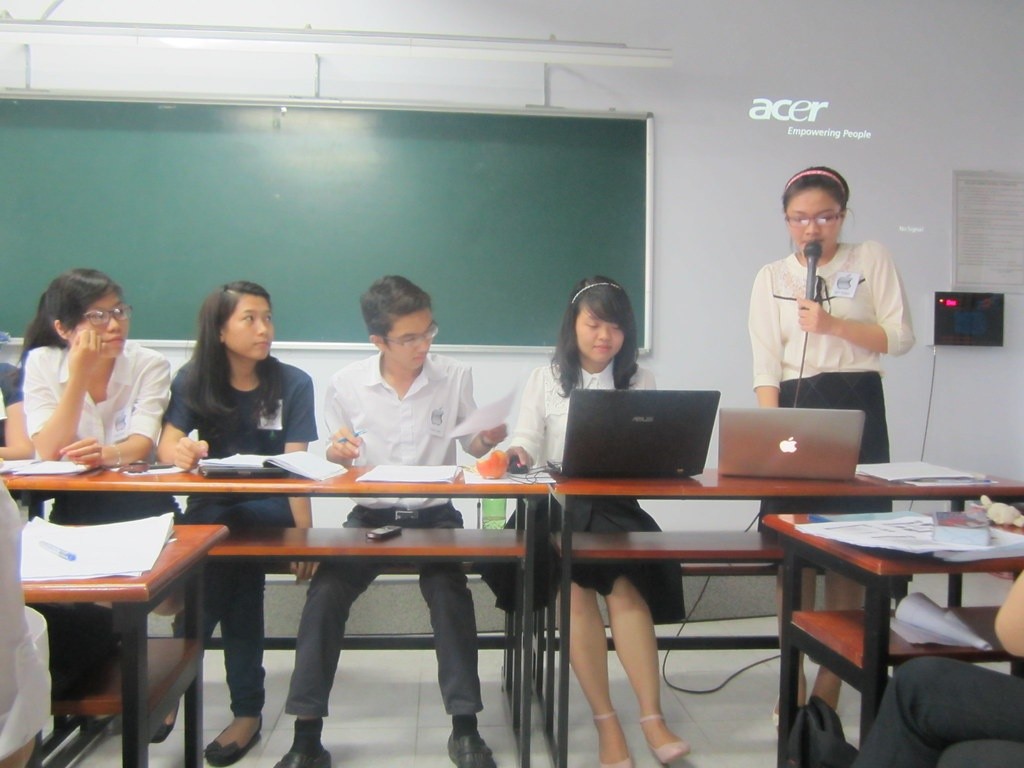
[377, 320, 440, 348]
[76, 303, 133, 327]
[785, 208, 844, 227]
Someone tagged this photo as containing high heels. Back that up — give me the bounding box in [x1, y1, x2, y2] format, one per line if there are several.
[591, 711, 633, 768]
[640, 714, 690, 768]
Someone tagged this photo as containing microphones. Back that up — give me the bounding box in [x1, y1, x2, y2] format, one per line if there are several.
[803, 241, 821, 310]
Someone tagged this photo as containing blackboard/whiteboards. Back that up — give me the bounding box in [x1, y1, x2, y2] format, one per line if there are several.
[0, 88, 653, 355]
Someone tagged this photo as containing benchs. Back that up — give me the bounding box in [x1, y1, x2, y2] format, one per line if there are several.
[204, 563, 513, 689]
[532, 563, 779, 693]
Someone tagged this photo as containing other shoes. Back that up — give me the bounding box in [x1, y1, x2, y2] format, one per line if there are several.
[152, 704, 179, 742]
[205, 711, 263, 765]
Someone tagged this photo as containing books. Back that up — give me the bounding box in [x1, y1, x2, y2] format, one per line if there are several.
[201, 451, 348, 481]
[808, 510, 1024, 561]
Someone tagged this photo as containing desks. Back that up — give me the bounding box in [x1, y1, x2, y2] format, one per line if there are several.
[0, 464, 1024, 768]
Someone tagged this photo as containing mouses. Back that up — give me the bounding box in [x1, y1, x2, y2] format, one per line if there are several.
[506, 455, 528, 473]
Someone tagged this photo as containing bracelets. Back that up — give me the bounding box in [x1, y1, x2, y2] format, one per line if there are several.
[480, 435, 495, 447]
[110, 444, 121, 467]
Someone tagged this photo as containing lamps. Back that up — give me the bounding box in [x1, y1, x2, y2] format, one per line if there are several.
[0, 19, 676, 72]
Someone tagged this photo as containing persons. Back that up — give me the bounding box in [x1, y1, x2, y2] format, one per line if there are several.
[750, 165, 916, 731]
[0, 267, 173, 768]
[850, 571, 1024, 768]
[273, 276, 507, 767]
[506, 277, 691, 768]
[151, 280, 319, 768]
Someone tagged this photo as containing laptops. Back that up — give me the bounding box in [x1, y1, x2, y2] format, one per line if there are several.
[718, 407, 865, 481]
[546, 388, 721, 476]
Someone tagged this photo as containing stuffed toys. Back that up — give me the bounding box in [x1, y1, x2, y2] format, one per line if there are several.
[981, 494, 1024, 527]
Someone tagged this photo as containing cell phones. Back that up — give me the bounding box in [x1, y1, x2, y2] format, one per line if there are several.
[368, 526, 402, 539]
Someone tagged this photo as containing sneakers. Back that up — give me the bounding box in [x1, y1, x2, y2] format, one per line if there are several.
[448, 730, 497, 768]
[275, 747, 331, 768]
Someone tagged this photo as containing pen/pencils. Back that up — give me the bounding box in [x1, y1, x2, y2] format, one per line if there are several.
[38, 541, 75, 561]
[337, 430, 366, 443]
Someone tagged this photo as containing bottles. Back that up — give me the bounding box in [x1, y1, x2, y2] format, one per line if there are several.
[481, 498, 507, 530]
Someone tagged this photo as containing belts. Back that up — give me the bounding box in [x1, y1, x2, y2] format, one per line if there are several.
[367, 507, 433, 521]
